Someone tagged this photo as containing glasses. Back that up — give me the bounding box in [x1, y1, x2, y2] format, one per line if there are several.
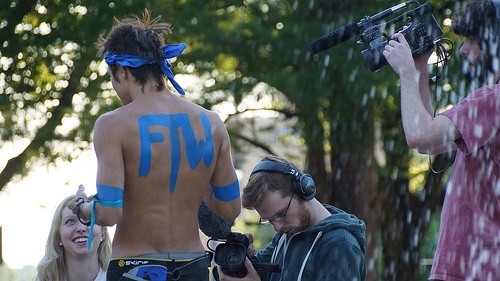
[259, 191, 295, 226]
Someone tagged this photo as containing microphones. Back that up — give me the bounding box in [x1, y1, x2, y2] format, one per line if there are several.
[311, 0, 417, 55]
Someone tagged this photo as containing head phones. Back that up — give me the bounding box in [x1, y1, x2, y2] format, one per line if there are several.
[251, 161, 317, 201]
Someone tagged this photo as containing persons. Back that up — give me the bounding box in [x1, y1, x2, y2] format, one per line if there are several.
[384, 0, 500, 281]
[68, 7, 241, 281]
[34, 195, 118, 281]
[217, 155, 369, 281]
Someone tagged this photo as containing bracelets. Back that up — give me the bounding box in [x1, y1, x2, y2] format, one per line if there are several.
[72, 197, 92, 227]
